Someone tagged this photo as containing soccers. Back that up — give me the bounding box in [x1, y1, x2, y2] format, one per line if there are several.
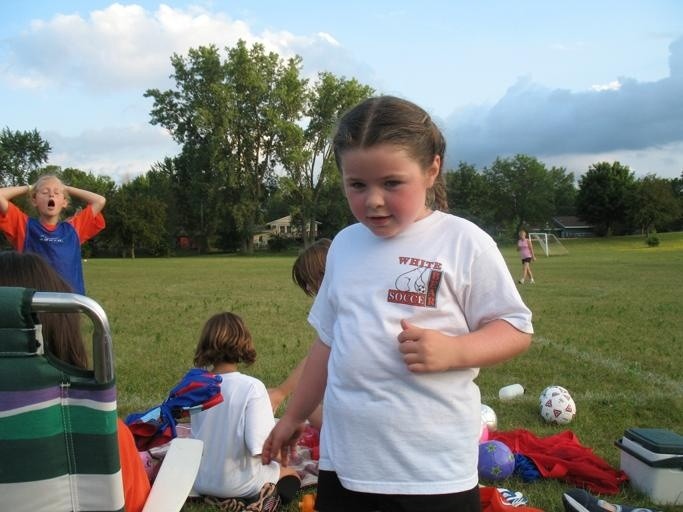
[477, 440, 515, 482]
[542, 392, 576, 424]
[480, 404, 498, 432]
[538, 386, 568, 407]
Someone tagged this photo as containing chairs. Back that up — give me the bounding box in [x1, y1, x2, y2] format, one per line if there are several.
[0, 286, 204, 512]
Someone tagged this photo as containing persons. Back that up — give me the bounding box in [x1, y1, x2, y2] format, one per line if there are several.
[0, 175, 107, 295]
[258, 95, 534, 512]
[515, 230, 536, 286]
[263, 236, 335, 430]
[0, 249, 151, 511]
[129, 309, 300, 512]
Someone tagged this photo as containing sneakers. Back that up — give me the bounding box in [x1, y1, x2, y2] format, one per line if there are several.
[559, 488, 665, 512]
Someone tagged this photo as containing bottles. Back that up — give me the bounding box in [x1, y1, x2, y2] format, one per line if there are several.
[498, 383, 527, 399]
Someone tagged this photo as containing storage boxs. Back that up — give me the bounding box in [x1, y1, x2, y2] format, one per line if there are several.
[614, 427, 683, 507]
